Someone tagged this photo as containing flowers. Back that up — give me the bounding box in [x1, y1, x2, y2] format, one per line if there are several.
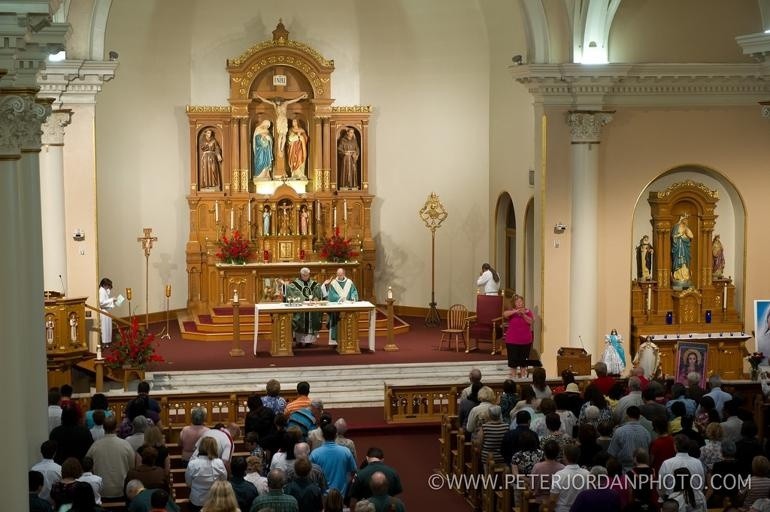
[102, 307, 166, 369]
[745, 352, 766, 366]
[318, 224, 360, 261]
[215, 227, 255, 265]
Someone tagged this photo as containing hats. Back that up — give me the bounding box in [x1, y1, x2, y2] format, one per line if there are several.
[564, 383, 582, 394]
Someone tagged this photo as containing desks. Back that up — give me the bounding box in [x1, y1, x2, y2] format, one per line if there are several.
[254, 302, 376, 358]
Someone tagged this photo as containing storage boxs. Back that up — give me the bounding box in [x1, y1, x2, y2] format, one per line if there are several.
[556, 347, 591, 376]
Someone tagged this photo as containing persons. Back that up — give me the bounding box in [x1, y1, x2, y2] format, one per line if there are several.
[327, 268, 362, 346]
[658, 435, 706, 496]
[299, 208, 308, 236]
[632, 336, 660, 383]
[459, 382, 487, 425]
[650, 380, 666, 394]
[184, 436, 229, 510]
[243, 433, 270, 461]
[126, 416, 147, 450]
[500, 410, 540, 462]
[324, 490, 346, 512]
[244, 456, 271, 495]
[337, 127, 362, 191]
[286, 118, 307, 178]
[712, 234, 727, 281]
[299, 485, 325, 512]
[528, 368, 551, 400]
[503, 294, 534, 378]
[579, 388, 614, 423]
[547, 443, 593, 512]
[671, 214, 696, 290]
[285, 381, 323, 416]
[533, 398, 577, 440]
[125, 382, 163, 418]
[307, 414, 333, 448]
[253, 93, 312, 178]
[570, 466, 621, 512]
[199, 480, 240, 512]
[650, 419, 679, 461]
[467, 386, 503, 432]
[177, 407, 212, 461]
[627, 366, 650, 390]
[459, 368, 483, 399]
[308, 424, 357, 496]
[272, 434, 304, 471]
[276, 267, 333, 348]
[511, 432, 545, 477]
[685, 374, 705, 403]
[198, 131, 222, 190]
[80, 460, 103, 507]
[589, 362, 617, 395]
[51, 404, 93, 464]
[681, 348, 702, 381]
[666, 382, 696, 430]
[601, 329, 628, 375]
[704, 424, 730, 465]
[46, 388, 63, 428]
[698, 396, 717, 426]
[476, 263, 502, 295]
[670, 400, 698, 433]
[353, 500, 376, 512]
[142, 491, 170, 512]
[597, 419, 615, 456]
[743, 452, 770, 512]
[642, 391, 667, 428]
[252, 119, 273, 183]
[61, 385, 79, 408]
[540, 412, 579, 452]
[128, 480, 183, 512]
[28, 441, 64, 502]
[286, 442, 328, 493]
[263, 208, 273, 235]
[659, 499, 681, 512]
[362, 472, 405, 512]
[720, 402, 746, 445]
[510, 384, 541, 417]
[86, 417, 132, 500]
[704, 373, 735, 416]
[190, 423, 240, 474]
[85, 393, 113, 435]
[136, 423, 171, 470]
[530, 441, 563, 494]
[98, 277, 117, 349]
[614, 379, 643, 411]
[52, 459, 92, 512]
[250, 468, 299, 512]
[28, 470, 50, 512]
[348, 447, 402, 497]
[243, 396, 278, 436]
[256, 380, 289, 414]
[285, 400, 322, 433]
[501, 379, 521, 409]
[91, 411, 106, 439]
[560, 370, 582, 395]
[582, 406, 606, 428]
[625, 447, 653, 499]
[615, 406, 651, 465]
[479, 408, 509, 462]
[333, 418, 359, 462]
[634, 235, 657, 282]
[670, 468, 710, 512]
[133, 396, 159, 421]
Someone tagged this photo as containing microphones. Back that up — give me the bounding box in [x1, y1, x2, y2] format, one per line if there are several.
[59, 275, 66, 298]
[579, 335, 585, 354]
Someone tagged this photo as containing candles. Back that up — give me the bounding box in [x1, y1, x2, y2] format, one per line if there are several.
[214, 199, 219, 220]
[344, 199, 347, 222]
[247, 200, 251, 222]
[646, 287, 652, 310]
[723, 282, 728, 308]
[317, 200, 320, 222]
[230, 209, 235, 227]
[333, 207, 337, 229]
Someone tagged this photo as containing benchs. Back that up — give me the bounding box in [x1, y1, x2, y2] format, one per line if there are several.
[439, 415, 770, 512]
[33, 442, 323, 508]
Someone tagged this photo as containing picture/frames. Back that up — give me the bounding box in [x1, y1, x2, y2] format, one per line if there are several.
[754, 299, 770, 354]
[674, 341, 710, 391]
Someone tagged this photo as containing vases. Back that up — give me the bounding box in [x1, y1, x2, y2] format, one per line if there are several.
[748, 368, 761, 380]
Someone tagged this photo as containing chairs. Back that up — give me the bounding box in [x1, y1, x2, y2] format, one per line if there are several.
[465, 288, 505, 356]
[437, 303, 468, 352]
